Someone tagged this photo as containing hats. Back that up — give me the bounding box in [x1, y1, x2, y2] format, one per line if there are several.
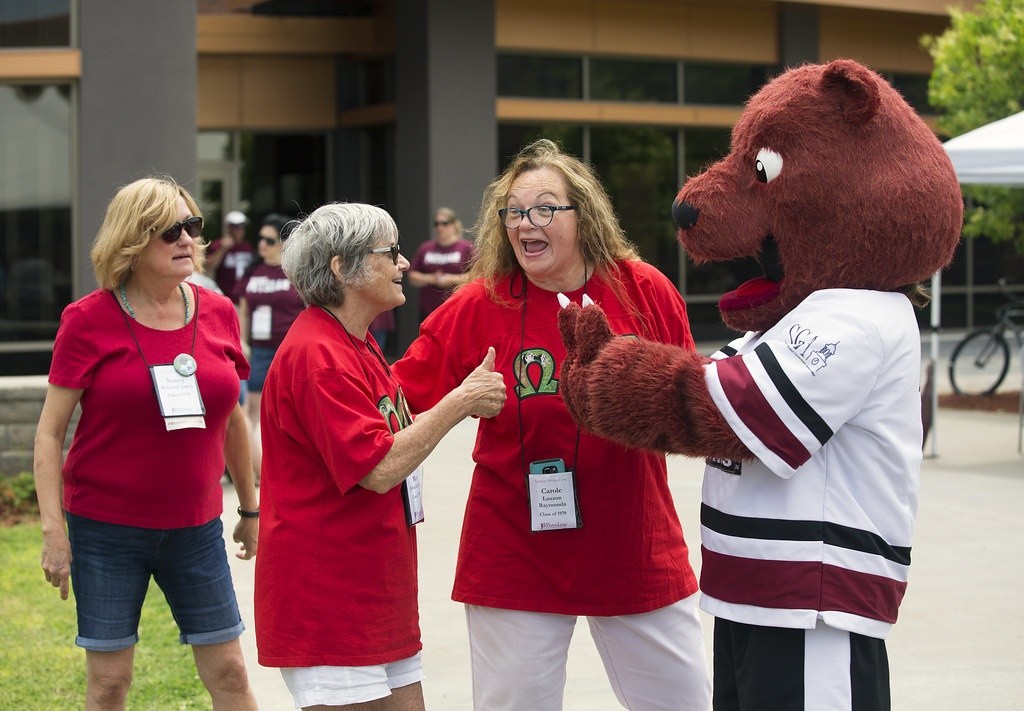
[225, 212, 247, 227]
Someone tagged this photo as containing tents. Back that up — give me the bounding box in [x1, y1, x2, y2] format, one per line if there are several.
[928, 106, 1024, 456]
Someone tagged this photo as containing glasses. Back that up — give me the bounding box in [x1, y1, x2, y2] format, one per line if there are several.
[257, 234, 283, 246]
[498, 205, 578, 229]
[434, 221, 453, 226]
[359, 245, 401, 266]
[148, 217, 203, 243]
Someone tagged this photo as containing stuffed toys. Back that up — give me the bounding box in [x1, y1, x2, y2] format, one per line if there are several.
[556, 58, 964, 711]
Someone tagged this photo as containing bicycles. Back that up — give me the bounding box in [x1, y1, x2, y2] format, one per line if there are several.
[949, 279, 1024, 399]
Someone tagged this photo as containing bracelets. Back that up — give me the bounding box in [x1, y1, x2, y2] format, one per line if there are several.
[237, 506, 260, 518]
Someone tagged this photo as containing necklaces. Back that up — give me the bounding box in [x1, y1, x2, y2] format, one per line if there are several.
[120, 283, 189, 328]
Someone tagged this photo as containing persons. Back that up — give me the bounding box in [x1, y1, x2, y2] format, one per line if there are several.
[369, 307, 395, 356]
[32, 178, 259, 711]
[185, 209, 308, 489]
[387, 139, 712, 711]
[408, 207, 480, 325]
[253, 204, 507, 711]
